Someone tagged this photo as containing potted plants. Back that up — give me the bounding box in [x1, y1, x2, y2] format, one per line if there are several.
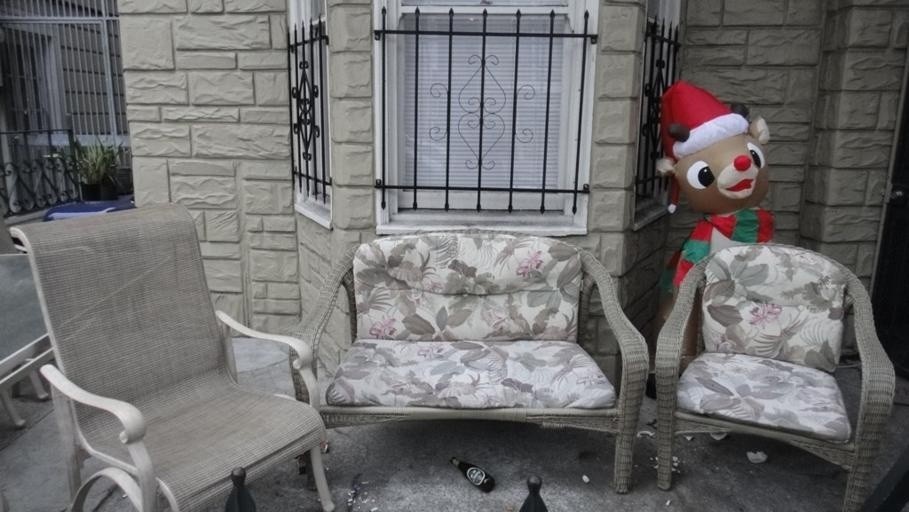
[94, 133, 133, 202]
[59, 128, 107, 201]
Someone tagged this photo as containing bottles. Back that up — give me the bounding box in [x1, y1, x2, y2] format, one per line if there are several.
[225, 465, 257, 511]
[519, 475, 548, 511]
[449, 455, 496, 494]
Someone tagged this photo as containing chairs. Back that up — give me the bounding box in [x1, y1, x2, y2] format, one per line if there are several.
[654, 240, 898, 511]
[7, 200, 337, 510]
[285, 225, 652, 495]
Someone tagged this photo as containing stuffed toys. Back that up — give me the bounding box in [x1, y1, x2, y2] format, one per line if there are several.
[655, 80, 776, 303]
